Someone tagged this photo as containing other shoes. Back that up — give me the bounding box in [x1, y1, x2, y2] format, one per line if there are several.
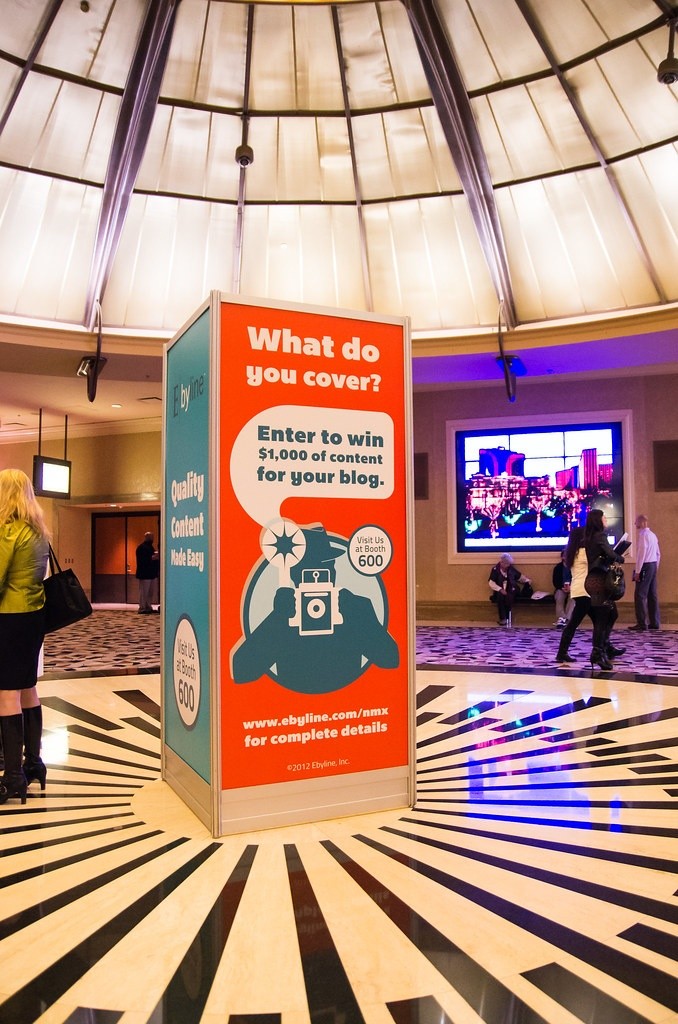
[138, 611, 151, 614]
[497, 619, 509, 625]
[553, 617, 567, 627]
[149, 608, 157, 613]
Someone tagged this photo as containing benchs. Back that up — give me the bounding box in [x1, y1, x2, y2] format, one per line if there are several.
[488, 593, 556, 625]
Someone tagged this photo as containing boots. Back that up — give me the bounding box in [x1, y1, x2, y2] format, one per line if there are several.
[0, 713, 28, 804]
[22, 704, 47, 790]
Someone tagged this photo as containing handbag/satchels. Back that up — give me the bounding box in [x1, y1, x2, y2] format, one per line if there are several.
[584, 557, 625, 601]
[44, 541, 93, 633]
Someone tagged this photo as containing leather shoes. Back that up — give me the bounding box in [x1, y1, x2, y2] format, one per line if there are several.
[647, 624, 659, 630]
[607, 647, 626, 660]
[628, 624, 647, 631]
[555, 653, 577, 663]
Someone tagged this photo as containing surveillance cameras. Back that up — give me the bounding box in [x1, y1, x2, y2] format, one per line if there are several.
[234, 146, 254, 167]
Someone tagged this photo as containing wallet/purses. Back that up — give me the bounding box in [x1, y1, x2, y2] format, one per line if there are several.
[632, 570, 645, 581]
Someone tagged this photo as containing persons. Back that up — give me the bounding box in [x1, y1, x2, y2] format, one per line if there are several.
[552, 509, 660, 670]
[135, 532, 159, 613]
[0, 468, 50, 805]
[488, 553, 532, 625]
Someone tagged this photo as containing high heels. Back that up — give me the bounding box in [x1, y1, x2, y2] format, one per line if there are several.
[590, 648, 613, 670]
